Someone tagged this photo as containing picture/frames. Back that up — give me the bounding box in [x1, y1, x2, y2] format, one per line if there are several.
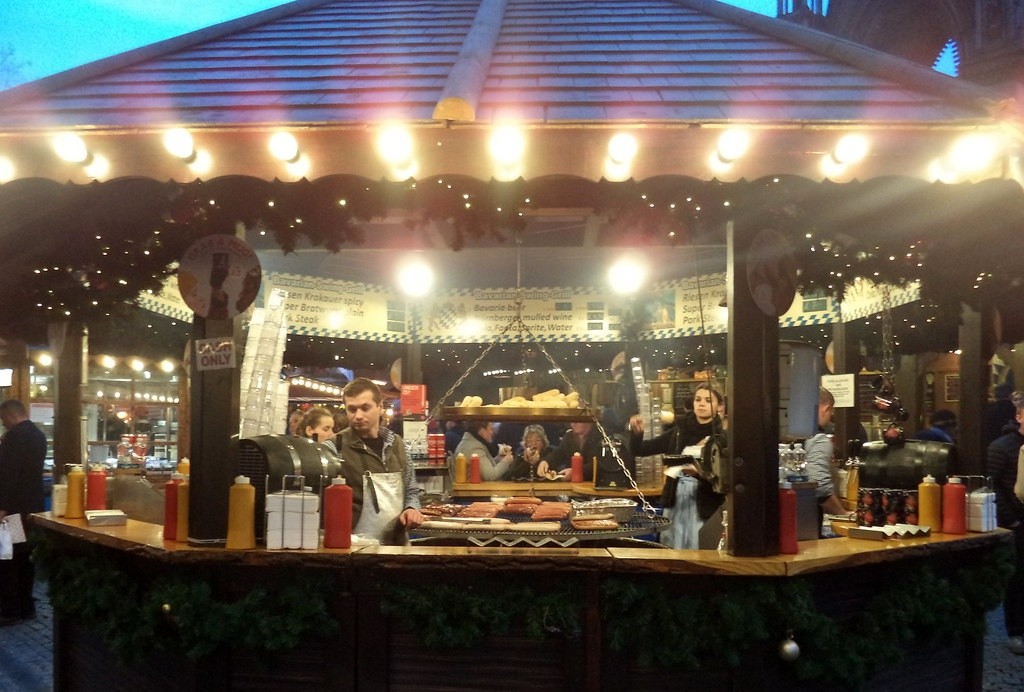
[942, 372, 962, 402]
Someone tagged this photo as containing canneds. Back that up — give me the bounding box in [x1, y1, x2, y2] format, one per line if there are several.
[427, 433, 445, 459]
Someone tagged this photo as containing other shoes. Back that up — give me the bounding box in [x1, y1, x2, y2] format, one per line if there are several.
[1007, 635, 1024, 655]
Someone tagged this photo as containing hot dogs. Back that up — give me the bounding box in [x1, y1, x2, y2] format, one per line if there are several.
[546, 470, 556, 479]
[499, 445, 511, 455]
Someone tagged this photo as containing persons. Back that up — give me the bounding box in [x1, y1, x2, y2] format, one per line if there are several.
[285, 377, 423, 545]
[0, 399, 48, 627]
[919, 383, 1024, 655]
[427, 418, 612, 480]
[779, 385, 858, 538]
[626, 382, 727, 551]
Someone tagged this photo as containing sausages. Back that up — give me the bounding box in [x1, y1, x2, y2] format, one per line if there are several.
[419, 496, 619, 532]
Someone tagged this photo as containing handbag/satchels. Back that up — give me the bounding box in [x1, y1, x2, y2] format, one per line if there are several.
[0, 520, 14, 560]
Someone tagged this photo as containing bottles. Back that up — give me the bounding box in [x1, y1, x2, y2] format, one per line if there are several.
[162, 457, 189, 542]
[225, 476, 256, 550]
[470, 454, 481, 484]
[324, 475, 351, 549]
[779, 480, 799, 554]
[571, 452, 583, 483]
[116, 433, 133, 469]
[63, 465, 86, 519]
[779, 442, 807, 482]
[454, 453, 467, 483]
[917, 474, 941, 533]
[133, 433, 150, 468]
[86, 464, 107, 510]
[941, 477, 966, 535]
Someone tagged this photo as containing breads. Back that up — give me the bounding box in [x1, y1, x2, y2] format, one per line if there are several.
[460, 389, 580, 409]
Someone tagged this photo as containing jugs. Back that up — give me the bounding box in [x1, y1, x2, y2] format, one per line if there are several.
[837, 457, 859, 500]
